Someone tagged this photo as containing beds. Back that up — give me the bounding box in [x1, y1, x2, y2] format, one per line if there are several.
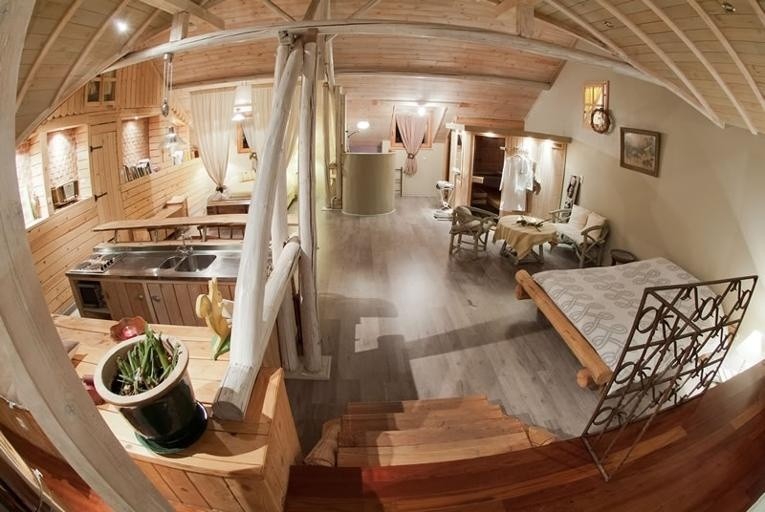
[515, 257, 728, 396]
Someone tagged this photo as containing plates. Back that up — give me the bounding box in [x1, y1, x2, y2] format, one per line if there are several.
[110, 316, 148, 340]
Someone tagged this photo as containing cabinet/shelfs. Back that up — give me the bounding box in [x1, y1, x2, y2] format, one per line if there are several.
[446, 123, 572, 227]
[100, 278, 236, 326]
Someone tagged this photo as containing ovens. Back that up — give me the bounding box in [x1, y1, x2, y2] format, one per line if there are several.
[75, 281, 103, 308]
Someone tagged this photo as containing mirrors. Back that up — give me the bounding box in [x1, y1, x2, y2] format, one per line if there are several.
[454, 133, 464, 175]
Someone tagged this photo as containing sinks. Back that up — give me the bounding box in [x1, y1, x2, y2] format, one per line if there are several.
[174, 254, 217, 271]
[159, 255, 183, 269]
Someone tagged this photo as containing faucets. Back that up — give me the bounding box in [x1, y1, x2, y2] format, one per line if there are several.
[180, 233, 187, 251]
[347, 130, 360, 138]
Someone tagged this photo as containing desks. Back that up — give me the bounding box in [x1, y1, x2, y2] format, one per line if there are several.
[611, 250, 638, 265]
[499, 215, 556, 266]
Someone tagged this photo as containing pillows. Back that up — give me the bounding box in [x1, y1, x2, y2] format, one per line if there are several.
[568, 204, 606, 239]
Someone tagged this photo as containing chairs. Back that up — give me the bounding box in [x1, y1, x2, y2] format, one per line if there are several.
[449, 206, 496, 260]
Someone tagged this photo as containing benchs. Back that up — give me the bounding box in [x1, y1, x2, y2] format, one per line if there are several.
[548, 209, 610, 268]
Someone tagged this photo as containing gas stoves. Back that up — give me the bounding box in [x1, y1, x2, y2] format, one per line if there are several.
[70, 252, 127, 274]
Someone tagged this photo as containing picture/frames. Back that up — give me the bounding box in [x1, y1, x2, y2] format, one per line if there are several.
[620, 127, 660, 177]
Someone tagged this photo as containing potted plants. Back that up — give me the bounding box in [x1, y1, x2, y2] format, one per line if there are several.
[94, 330, 200, 445]
